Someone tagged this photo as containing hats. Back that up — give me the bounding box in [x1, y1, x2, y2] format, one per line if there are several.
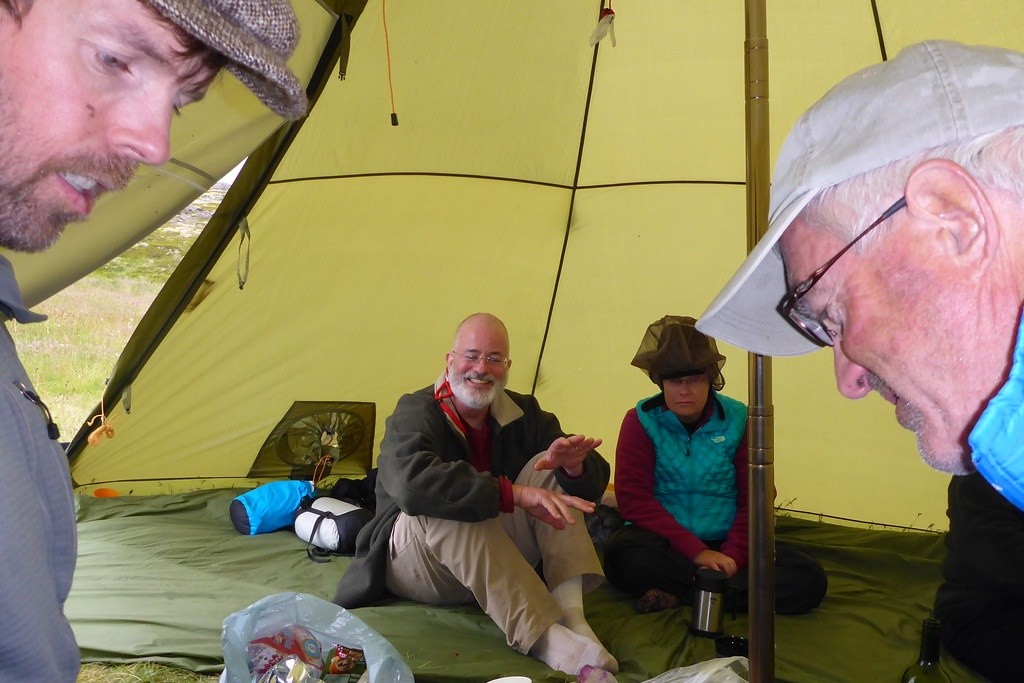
[694, 40, 1024, 356]
[145, 0, 309, 121]
[630, 314, 726, 391]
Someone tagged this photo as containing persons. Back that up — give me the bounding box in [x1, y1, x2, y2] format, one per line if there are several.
[1, 1, 310, 683]
[330, 312, 620, 675]
[694, 39, 1023, 683]
[603, 315, 827, 618]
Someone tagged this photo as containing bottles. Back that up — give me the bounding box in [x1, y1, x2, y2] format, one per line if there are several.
[901, 619, 951, 683]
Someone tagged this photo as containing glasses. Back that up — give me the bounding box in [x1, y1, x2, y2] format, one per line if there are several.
[775, 196, 910, 346]
[663, 377, 710, 385]
[452, 351, 509, 369]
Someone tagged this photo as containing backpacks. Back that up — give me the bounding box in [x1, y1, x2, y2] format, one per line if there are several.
[294, 467, 381, 563]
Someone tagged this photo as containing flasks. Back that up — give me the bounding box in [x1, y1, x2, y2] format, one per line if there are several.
[690, 585, 722, 639]
